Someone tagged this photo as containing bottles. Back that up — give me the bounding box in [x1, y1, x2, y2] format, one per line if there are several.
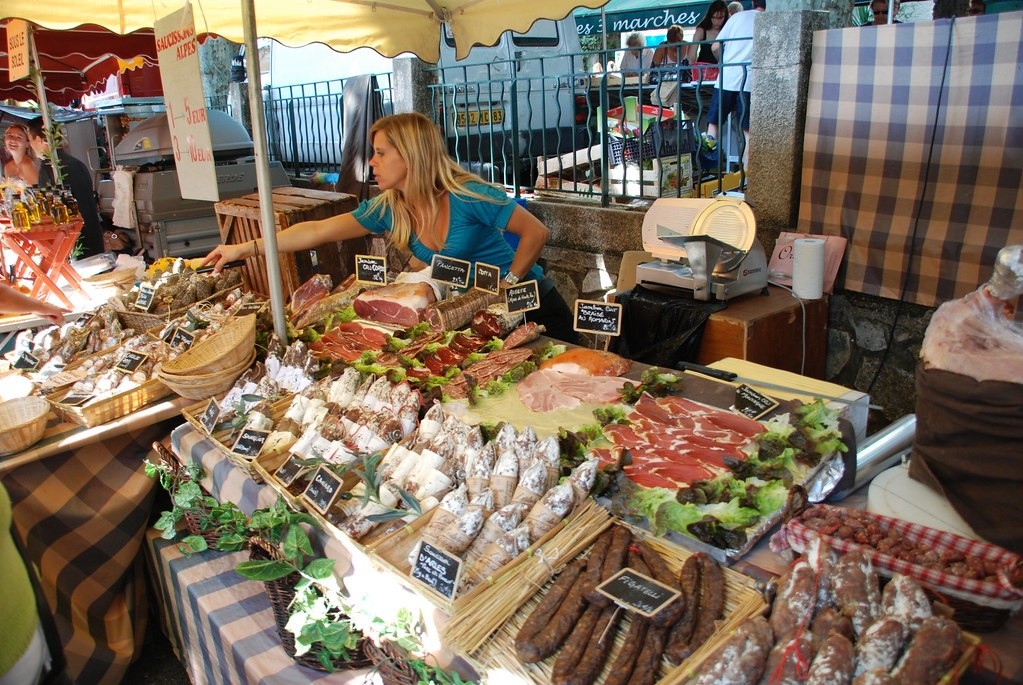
[0, 178, 78, 231]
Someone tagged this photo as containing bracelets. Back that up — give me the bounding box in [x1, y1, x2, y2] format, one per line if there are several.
[254, 239, 259, 256]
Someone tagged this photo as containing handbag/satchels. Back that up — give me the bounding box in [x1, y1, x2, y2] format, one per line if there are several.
[649, 59, 692, 83]
[691, 60, 719, 80]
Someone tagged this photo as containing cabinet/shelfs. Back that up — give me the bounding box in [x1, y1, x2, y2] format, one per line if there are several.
[609, 286, 828, 382]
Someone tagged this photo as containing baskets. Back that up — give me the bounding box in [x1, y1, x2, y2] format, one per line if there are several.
[0, 281, 276, 461]
[180, 371, 586, 609]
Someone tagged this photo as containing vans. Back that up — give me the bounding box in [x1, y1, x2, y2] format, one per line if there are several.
[225, 10, 592, 195]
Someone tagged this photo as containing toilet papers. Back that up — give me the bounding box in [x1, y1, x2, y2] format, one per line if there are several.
[791, 238, 824, 300]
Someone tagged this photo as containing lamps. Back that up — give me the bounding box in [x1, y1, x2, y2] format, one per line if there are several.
[231, 55, 247, 83]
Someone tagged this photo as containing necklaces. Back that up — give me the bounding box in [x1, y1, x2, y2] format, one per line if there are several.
[12, 162, 23, 172]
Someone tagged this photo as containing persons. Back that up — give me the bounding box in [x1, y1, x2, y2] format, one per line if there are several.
[27, 116, 105, 261]
[859, 0, 985, 27]
[622, 2, 744, 83]
[3, 124, 41, 189]
[198, 112, 583, 349]
[705, 0, 766, 183]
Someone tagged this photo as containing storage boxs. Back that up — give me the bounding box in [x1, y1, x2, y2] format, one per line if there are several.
[534, 96, 748, 198]
[585, 74, 649, 87]
[685, 356, 871, 446]
[214, 186, 366, 304]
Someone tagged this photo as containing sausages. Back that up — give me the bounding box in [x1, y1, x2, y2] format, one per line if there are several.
[213, 333, 964, 685]
[13, 257, 271, 402]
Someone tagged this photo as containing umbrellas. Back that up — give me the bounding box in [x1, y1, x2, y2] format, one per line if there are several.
[0, 0, 607, 354]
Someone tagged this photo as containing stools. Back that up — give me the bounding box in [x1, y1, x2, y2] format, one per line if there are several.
[1, 209, 84, 310]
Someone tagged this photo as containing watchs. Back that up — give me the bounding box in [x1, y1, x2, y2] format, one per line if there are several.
[505, 272, 522, 285]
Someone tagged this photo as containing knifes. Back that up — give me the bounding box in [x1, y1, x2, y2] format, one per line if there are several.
[151, 260, 247, 280]
